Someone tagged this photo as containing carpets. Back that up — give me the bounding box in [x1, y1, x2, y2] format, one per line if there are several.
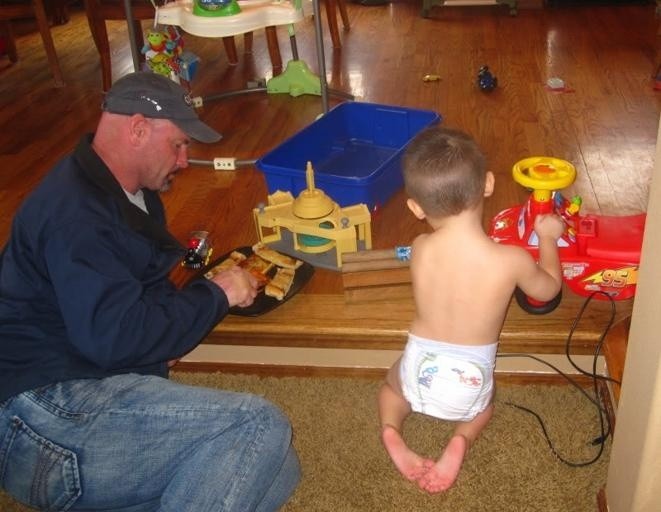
[0, 371, 613, 511]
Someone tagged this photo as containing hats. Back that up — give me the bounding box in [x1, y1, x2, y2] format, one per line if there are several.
[101, 70, 223, 146]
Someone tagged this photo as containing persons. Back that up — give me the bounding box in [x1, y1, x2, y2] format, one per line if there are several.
[1, 68, 306, 511]
[375, 124, 568, 494]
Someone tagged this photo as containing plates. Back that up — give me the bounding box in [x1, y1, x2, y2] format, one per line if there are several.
[188, 245, 315, 318]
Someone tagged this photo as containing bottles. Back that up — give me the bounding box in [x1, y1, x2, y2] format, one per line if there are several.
[546, 28, 568, 92]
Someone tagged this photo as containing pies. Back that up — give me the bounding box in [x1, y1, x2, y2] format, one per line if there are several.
[202, 243, 303, 302]
